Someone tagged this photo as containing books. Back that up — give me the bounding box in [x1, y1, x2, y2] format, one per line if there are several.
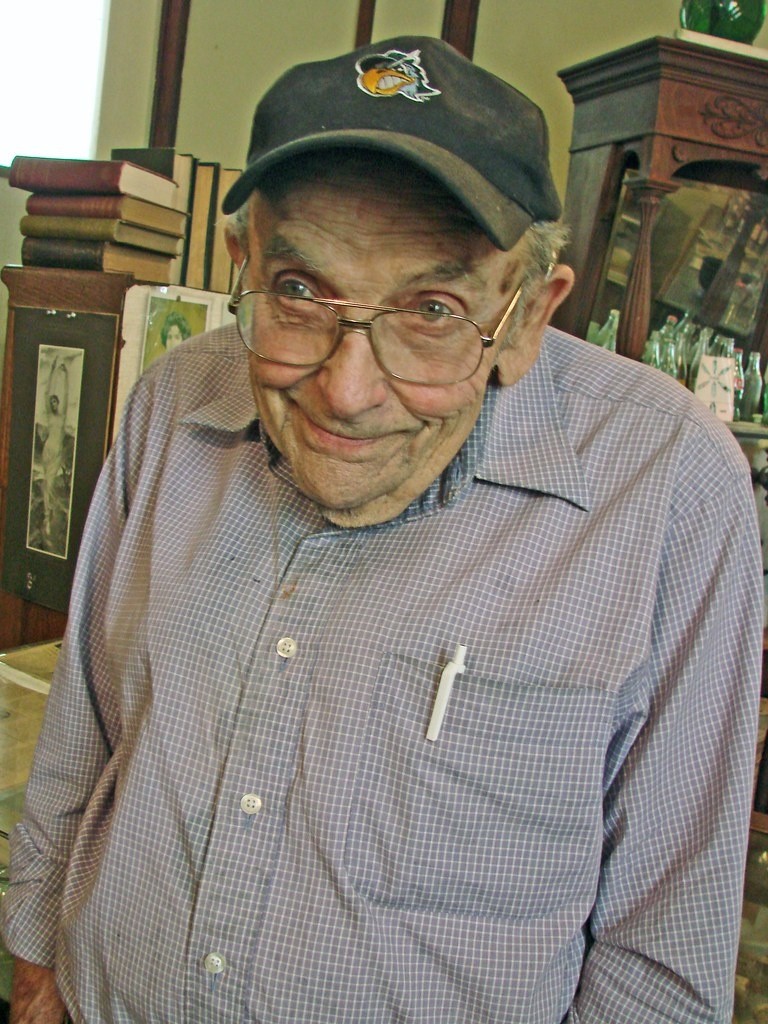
[9, 137, 248, 296]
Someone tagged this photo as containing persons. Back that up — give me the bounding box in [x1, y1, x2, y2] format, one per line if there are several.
[3, 33, 768, 1024]
[35, 341, 72, 556]
[158, 311, 195, 354]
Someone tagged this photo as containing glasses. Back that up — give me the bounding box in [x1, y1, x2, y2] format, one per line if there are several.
[228, 244, 531, 386]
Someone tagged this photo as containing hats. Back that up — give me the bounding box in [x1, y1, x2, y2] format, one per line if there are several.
[222, 35, 562, 252]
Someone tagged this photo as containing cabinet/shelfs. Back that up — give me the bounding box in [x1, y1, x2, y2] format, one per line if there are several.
[547, 34, 768, 835]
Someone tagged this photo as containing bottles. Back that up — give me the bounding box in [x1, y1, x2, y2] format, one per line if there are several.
[591, 309, 768, 425]
